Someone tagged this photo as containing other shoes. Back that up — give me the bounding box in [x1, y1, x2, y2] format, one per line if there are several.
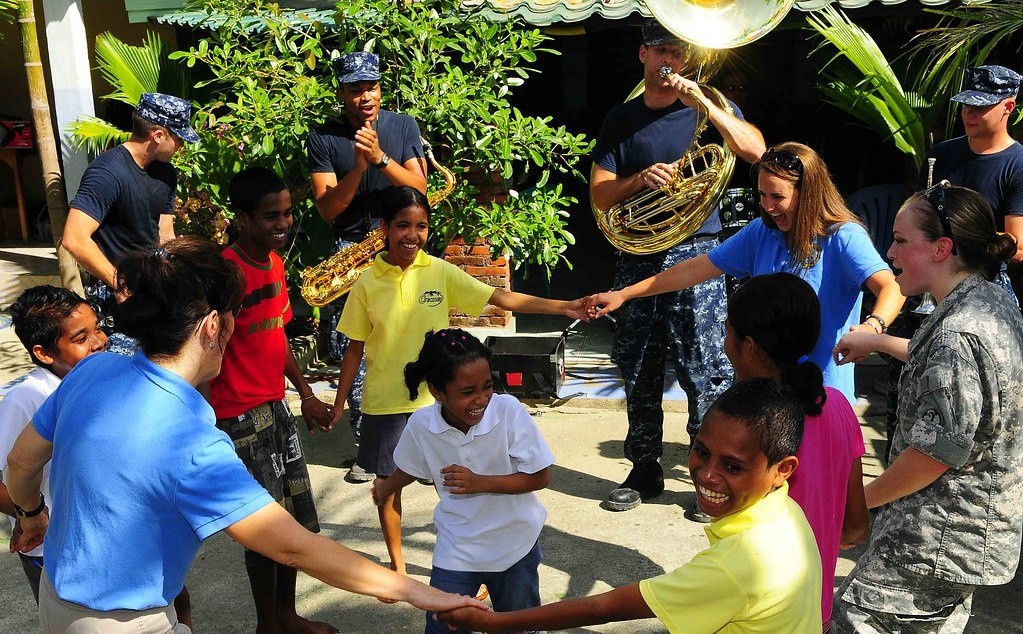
[347, 463, 377, 481]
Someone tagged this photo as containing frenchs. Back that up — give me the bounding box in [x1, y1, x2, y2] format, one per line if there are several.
[587, 0, 796, 257]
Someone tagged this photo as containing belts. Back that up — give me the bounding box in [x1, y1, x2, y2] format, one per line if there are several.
[341, 231, 366, 242]
[681, 233, 718, 245]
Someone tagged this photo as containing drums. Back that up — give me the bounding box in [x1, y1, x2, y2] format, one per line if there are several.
[717, 187, 759, 237]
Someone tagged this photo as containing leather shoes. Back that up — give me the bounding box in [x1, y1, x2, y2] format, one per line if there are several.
[606, 461, 664, 511]
[691, 499, 712, 523]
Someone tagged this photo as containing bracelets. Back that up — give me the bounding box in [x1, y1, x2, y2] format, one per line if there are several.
[866, 314, 887, 334]
[12, 491, 45, 518]
[300, 393, 315, 400]
[862, 322, 880, 334]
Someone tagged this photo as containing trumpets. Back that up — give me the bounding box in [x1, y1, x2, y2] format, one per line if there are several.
[911, 157, 955, 317]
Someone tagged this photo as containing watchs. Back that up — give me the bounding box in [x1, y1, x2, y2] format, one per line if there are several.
[374, 154, 390, 169]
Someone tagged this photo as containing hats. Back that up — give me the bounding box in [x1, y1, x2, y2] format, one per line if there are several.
[134, 93, 202, 143]
[640, 18, 680, 46]
[950, 65, 1023, 106]
[332, 52, 382, 83]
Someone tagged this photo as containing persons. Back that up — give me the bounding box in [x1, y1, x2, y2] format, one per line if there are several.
[724, 272, 869, 634]
[590, 18, 767, 523]
[0, 285, 193, 634]
[195, 164, 340, 634]
[830, 178, 1023, 634]
[920, 65, 1023, 311]
[371, 327, 556, 634]
[579, 141, 908, 406]
[60, 92, 202, 356]
[430, 375, 823, 634]
[320, 186, 589, 604]
[6, 233, 494, 634]
[307, 51, 434, 484]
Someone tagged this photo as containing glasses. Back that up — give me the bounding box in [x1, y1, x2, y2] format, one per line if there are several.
[194, 304, 243, 335]
[924, 179, 958, 256]
[761, 147, 803, 172]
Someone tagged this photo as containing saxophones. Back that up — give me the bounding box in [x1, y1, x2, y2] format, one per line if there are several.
[299, 134, 459, 309]
